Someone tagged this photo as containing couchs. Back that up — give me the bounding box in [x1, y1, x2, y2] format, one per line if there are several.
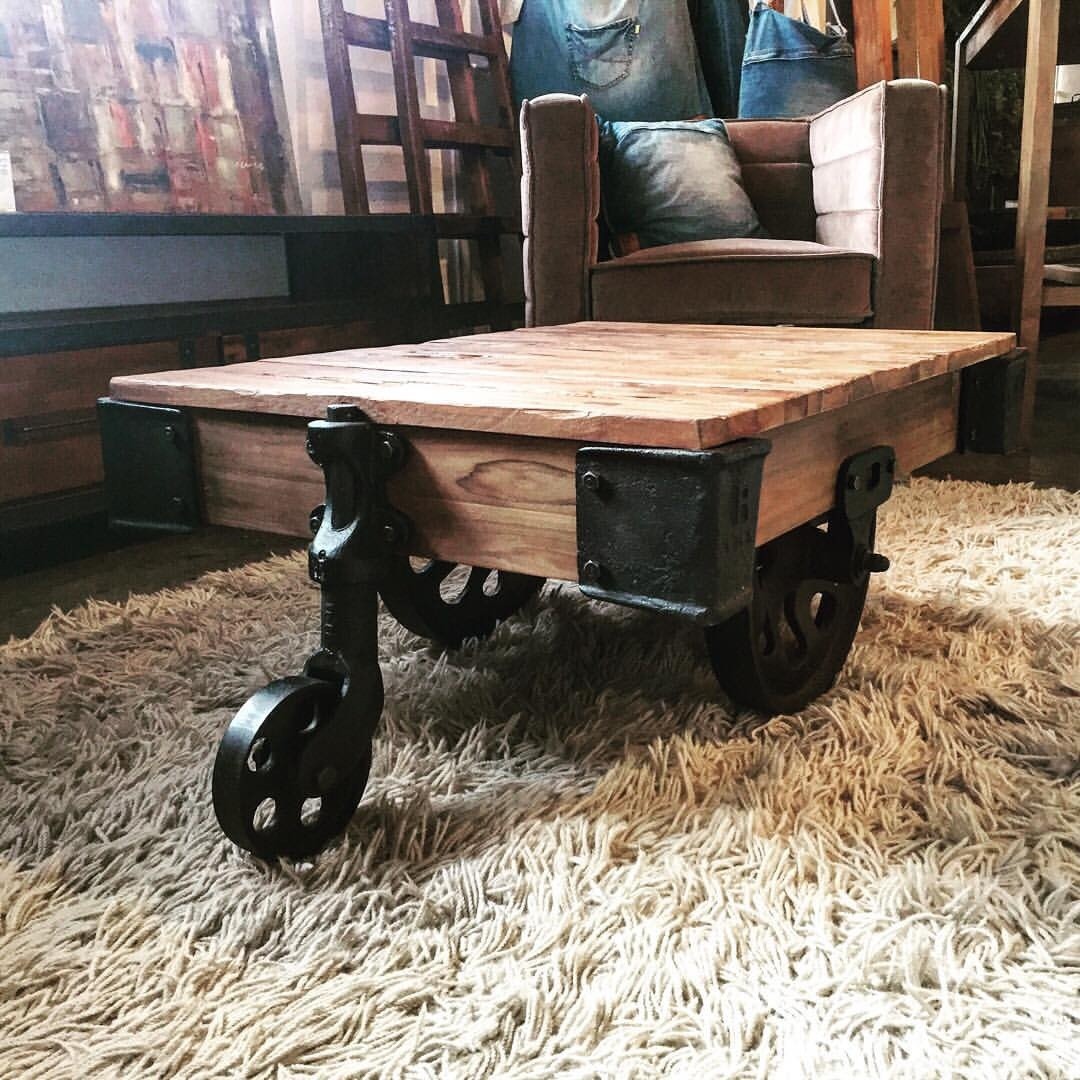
[519, 77, 947, 332]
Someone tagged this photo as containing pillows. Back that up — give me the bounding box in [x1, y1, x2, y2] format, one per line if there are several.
[594, 113, 772, 260]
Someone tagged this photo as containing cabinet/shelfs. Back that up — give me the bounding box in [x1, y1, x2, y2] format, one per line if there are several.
[947, 0, 1080, 462]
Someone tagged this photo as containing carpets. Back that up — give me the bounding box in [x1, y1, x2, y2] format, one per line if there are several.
[0, 471, 1080, 1079]
